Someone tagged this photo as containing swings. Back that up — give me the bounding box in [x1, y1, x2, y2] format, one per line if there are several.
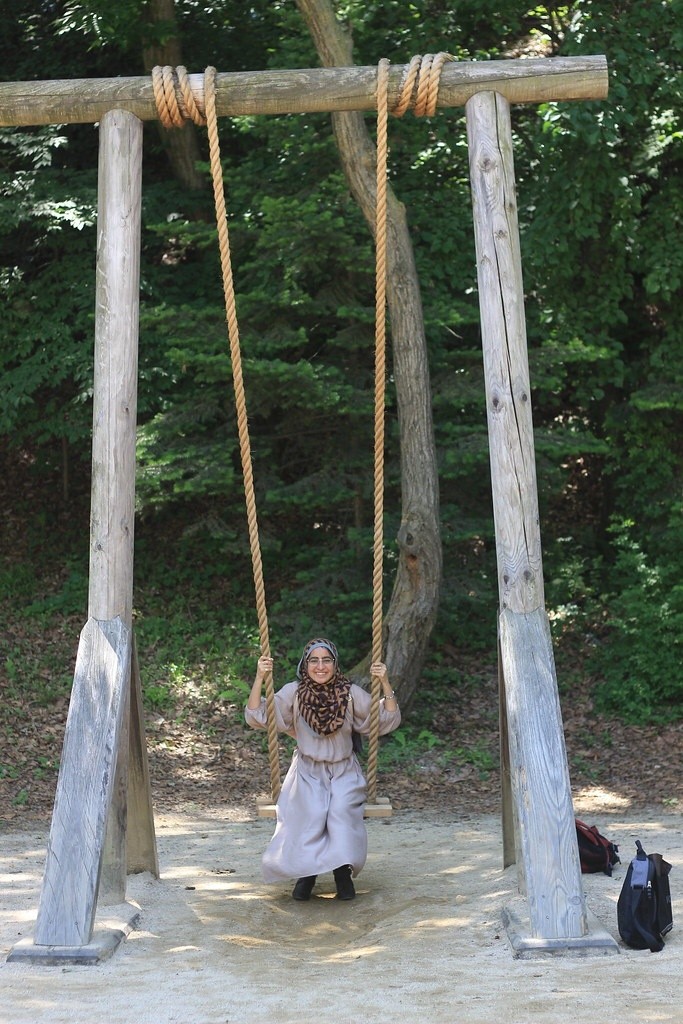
[204, 109, 394, 818]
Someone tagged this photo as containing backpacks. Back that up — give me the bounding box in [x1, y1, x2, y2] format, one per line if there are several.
[575, 819, 621, 877]
[617, 840, 673, 952]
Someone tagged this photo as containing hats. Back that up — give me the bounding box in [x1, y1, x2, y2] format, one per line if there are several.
[306, 642, 335, 658]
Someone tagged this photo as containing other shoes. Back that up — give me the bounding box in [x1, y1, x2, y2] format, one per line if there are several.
[332, 867, 355, 899]
[292, 875, 317, 900]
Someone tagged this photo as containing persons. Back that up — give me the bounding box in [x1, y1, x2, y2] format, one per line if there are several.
[245, 639, 400, 901]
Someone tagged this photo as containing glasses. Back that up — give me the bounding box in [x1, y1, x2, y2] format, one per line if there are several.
[307, 657, 335, 665]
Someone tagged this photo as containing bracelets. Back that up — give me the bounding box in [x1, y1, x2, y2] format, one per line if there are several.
[384, 690, 395, 699]
